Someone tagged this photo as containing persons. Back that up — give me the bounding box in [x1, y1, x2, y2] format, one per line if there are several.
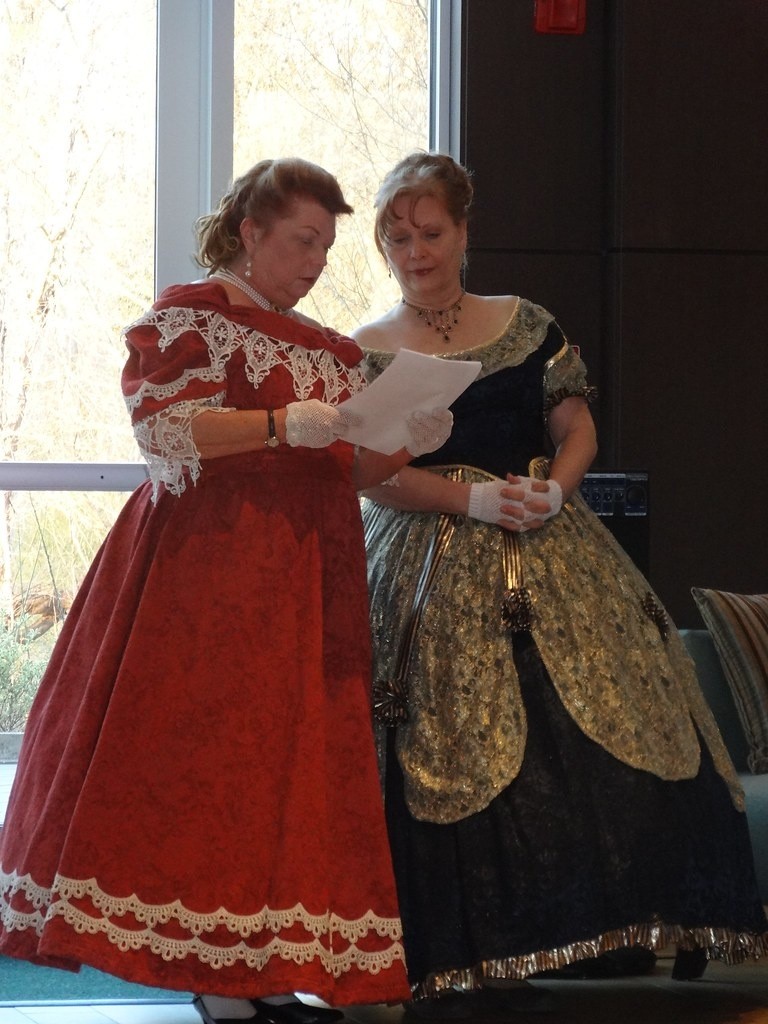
[352, 151, 768, 1024]
[0, 157, 448, 1024]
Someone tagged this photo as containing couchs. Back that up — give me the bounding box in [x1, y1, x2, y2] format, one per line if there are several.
[677, 628, 768, 906]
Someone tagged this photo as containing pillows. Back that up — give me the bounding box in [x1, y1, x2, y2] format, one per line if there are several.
[691, 587, 768, 774]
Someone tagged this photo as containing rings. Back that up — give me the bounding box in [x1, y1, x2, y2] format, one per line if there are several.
[431, 438, 439, 445]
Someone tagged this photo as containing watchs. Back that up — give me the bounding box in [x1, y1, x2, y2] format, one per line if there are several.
[265, 409, 279, 448]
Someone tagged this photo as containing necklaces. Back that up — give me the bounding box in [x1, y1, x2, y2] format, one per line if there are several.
[210, 267, 294, 319]
[402, 288, 466, 344]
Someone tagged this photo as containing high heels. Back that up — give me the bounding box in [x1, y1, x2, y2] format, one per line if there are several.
[252, 999, 344, 1024]
[190, 993, 273, 1024]
